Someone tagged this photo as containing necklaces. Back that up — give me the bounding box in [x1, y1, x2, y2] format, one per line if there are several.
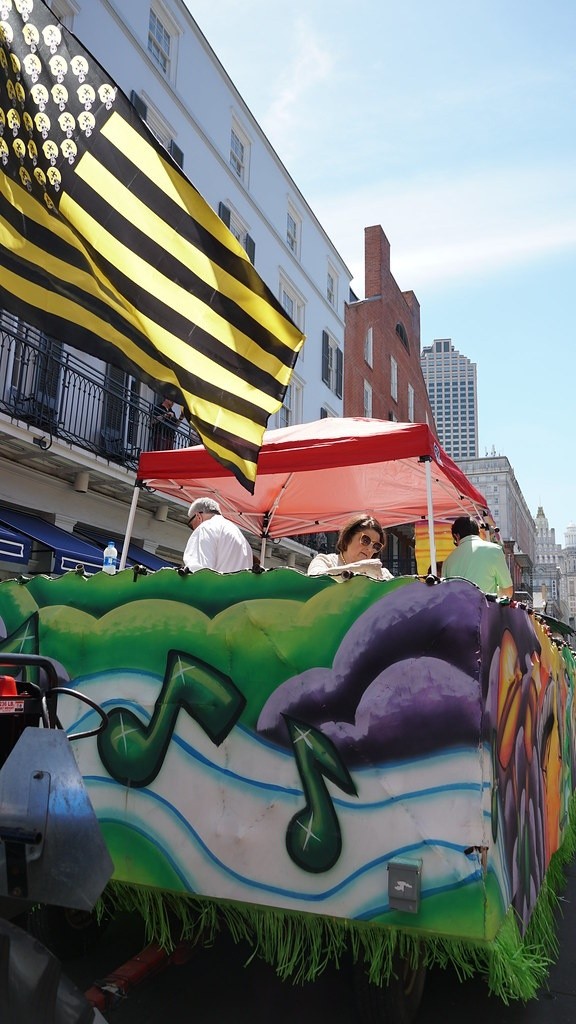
[340, 553, 347, 565]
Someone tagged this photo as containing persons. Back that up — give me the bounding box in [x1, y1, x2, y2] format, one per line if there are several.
[307, 513, 393, 578]
[153, 397, 177, 450]
[183, 498, 253, 572]
[441, 516, 513, 598]
[427, 562, 444, 577]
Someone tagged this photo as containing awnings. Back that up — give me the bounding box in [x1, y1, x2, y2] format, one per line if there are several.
[0, 503, 132, 578]
[0, 527, 32, 576]
[76, 526, 175, 575]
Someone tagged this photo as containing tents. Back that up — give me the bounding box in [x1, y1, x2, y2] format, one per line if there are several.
[118, 416, 500, 579]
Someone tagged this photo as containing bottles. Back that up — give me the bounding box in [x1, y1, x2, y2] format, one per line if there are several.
[102, 542, 117, 575]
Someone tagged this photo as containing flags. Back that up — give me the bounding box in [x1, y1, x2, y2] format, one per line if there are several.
[0, 1, 306, 495]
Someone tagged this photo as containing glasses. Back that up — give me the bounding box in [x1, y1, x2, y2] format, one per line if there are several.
[360, 531, 383, 553]
[186, 512, 203, 530]
[454, 537, 458, 546]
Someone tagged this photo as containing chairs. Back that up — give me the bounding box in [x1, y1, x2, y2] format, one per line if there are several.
[10, 386, 58, 435]
[101, 427, 142, 462]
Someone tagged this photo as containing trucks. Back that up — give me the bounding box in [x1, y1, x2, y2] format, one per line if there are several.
[0, 419, 576, 1024]
